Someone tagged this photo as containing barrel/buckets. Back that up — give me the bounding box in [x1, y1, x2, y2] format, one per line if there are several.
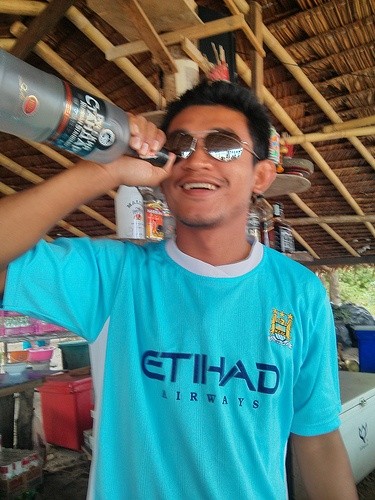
[354, 325, 375, 374]
[7, 351, 29, 364]
[56, 341, 90, 371]
[175, 59, 199, 97]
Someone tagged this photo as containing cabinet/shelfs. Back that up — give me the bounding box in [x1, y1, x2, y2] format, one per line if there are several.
[0, 332, 78, 374]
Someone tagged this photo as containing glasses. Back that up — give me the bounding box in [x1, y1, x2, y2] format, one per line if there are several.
[162, 130, 261, 161]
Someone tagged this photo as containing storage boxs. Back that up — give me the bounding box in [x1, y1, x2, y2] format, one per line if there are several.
[58, 340, 90, 370]
[0, 448, 42, 496]
[37, 373, 93, 451]
[350, 326, 375, 373]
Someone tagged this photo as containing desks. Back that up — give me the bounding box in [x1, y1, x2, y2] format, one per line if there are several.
[0, 378, 44, 451]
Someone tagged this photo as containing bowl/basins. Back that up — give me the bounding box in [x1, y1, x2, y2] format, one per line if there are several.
[1, 364, 28, 375]
[25, 347, 55, 361]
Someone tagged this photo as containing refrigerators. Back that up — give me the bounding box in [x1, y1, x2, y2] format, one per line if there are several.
[292, 369, 375, 500]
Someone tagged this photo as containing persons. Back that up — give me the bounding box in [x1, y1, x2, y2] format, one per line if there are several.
[0, 81, 359, 500]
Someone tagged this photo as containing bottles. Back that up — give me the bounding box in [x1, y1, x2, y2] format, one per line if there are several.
[154, 188, 176, 240]
[268, 121, 293, 172]
[114, 185, 145, 240]
[248, 200, 295, 254]
[138, 186, 164, 241]
[0, 47, 181, 166]
